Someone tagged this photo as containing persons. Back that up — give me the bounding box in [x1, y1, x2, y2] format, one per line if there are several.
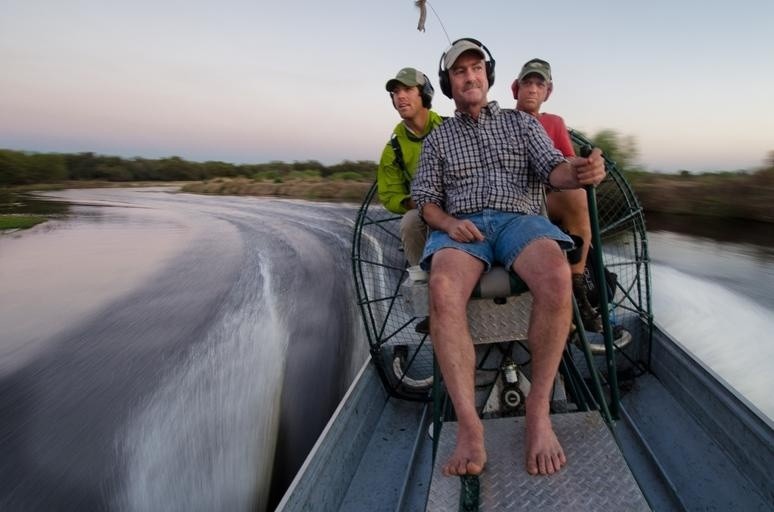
[377, 68, 447, 337]
[512, 57, 608, 355]
[410, 36, 608, 477]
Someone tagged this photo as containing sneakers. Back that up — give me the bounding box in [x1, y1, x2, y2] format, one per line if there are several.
[574, 275, 607, 327]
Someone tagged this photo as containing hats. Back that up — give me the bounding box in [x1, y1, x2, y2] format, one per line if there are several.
[518, 57, 553, 81]
[442, 39, 485, 69]
[385, 66, 435, 98]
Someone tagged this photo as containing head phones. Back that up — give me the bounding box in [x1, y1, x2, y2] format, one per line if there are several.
[439, 37, 495, 98]
[389, 73, 434, 108]
[511, 57, 553, 101]
